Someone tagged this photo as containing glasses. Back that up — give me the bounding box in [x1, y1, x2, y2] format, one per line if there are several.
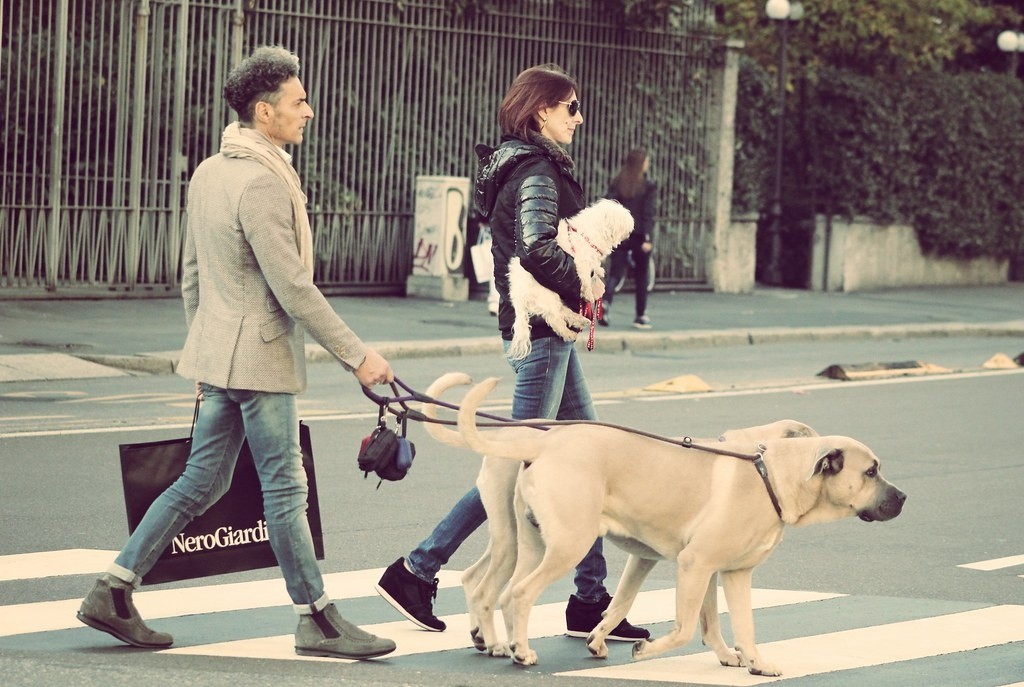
[556, 99, 581, 117]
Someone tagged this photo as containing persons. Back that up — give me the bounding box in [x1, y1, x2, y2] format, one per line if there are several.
[76, 47, 397, 661]
[595, 148, 654, 329]
[377, 67, 651, 642]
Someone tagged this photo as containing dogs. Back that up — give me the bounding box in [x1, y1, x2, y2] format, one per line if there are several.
[420, 371, 906, 676]
[505, 196, 636, 360]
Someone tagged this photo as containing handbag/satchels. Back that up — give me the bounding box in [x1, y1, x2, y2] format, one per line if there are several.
[470, 228, 494, 284]
[118, 393, 325, 586]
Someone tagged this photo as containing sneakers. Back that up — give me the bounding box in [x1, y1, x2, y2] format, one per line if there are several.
[375, 557, 447, 632]
[566, 594, 651, 641]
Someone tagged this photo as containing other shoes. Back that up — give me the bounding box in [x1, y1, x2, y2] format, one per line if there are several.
[633, 317, 653, 328]
[76, 575, 173, 648]
[295, 604, 395, 659]
[596, 303, 610, 326]
